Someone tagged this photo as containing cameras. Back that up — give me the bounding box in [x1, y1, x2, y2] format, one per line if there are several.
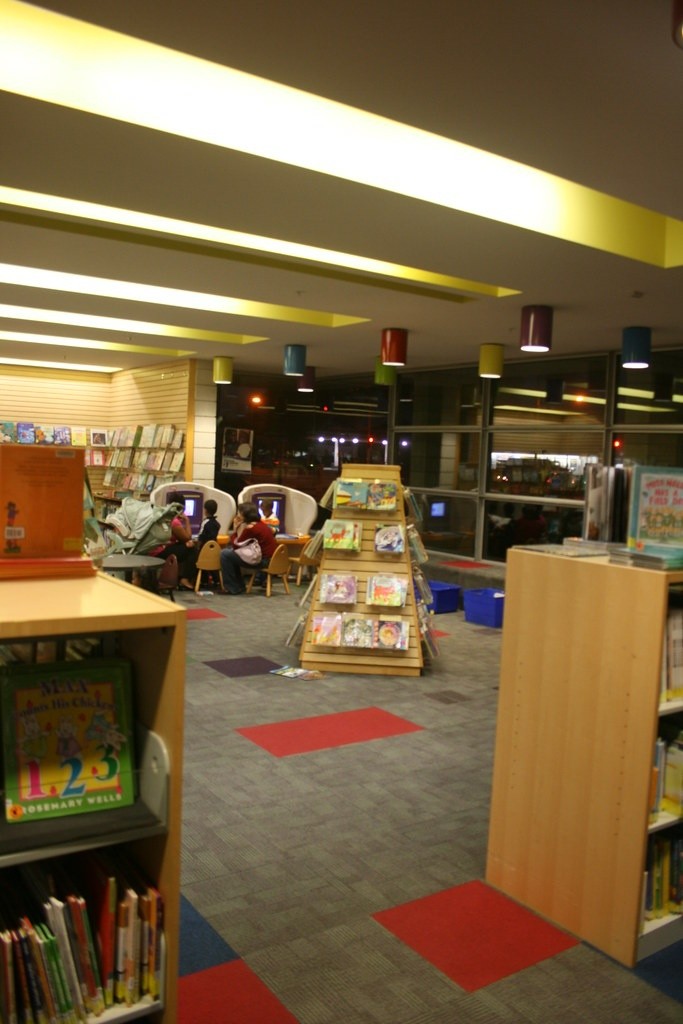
[431, 502, 445, 517]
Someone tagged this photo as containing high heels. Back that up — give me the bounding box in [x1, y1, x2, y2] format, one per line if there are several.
[177, 584, 194, 591]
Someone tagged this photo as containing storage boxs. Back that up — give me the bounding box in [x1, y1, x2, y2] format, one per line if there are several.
[461, 587, 505, 629]
[412, 581, 463, 614]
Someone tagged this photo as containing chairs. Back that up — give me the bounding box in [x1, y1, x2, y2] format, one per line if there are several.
[194, 540, 225, 593]
[157, 555, 181, 603]
[287, 539, 319, 587]
[245, 544, 291, 596]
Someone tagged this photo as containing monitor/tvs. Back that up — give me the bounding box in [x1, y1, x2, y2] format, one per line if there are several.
[183, 498, 195, 516]
[257, 497, 278, 517]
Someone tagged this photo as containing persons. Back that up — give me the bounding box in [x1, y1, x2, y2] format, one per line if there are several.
[260, 499, 280, 538]
[148, 492, 195, 591]
[215, 500, 278, 595]
[193, 500, 220, 587]
[488, 503, 545, 562]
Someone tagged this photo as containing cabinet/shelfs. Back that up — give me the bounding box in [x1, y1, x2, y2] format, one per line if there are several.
[0, 564, 184, 1024]
[91, 490, 150, 553]
[485, 536, 683, 969]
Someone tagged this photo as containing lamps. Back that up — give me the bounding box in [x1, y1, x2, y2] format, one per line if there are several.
[284, 345, 306, 377]
[477, 344, 503, 378]
[620, 326, 652, 369]
[380, 327, 409, 367]
[519, 305, 554, 353]
[374, 355, 396, 385]
[296, 367, 317, 392]
[213, 356, 234, 384]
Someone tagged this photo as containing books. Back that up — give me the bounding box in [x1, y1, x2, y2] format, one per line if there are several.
[106, 425, 186, 449]
[287, 614, 307, 648]
[509, 459, 683, 572]
[105, 447, 184, 472]
[0, 421, 108, 446]
[310, 613, 440, 657]
[0, 661, 139, 823]
[660, 608, 683, 704]
[271, 666, 306, 678]
[304, 519, 429, 564]
[638, 835, 683, 934]
[298, 572, 433, 608]
[318, 478, 424, 523]
[103, 470, 176, 492]
[85, 448, 105, 465]
[649, 728, 683, 823]
[0, 853, 165, 1024]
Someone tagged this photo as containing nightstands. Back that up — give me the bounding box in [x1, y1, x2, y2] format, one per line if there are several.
[185, 533, 310, 582]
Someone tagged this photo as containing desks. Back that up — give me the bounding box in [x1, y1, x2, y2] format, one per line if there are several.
[102, 554, 166, 584]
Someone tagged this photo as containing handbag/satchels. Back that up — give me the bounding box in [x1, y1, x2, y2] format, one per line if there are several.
[234, 542, 262, 566]
